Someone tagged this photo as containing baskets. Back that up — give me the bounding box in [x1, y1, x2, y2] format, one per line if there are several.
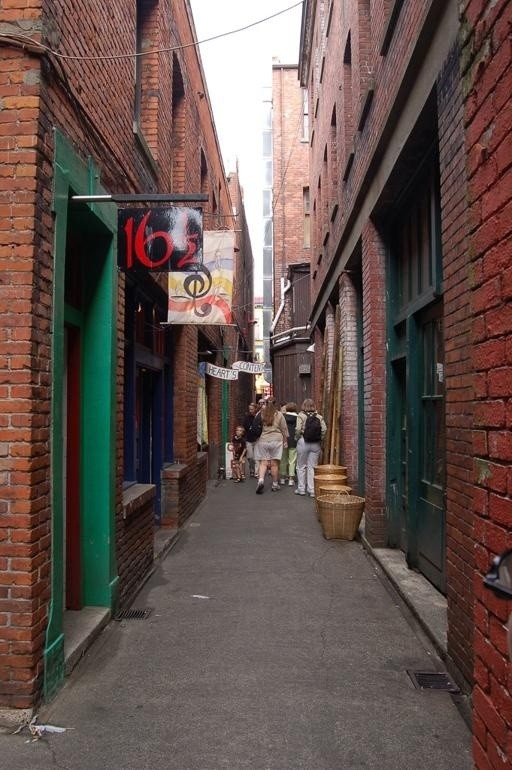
[313, 464, 366, 542]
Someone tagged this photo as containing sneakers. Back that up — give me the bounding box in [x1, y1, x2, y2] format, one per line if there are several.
[234, 471, 316, 498]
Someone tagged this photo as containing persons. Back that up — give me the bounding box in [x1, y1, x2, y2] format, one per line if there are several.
[247, 397, 291, 495]
[292, 398, 328, 499]
[227, 398, 300, 486]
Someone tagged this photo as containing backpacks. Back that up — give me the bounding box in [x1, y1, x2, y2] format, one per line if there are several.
[246, 411, 264, 443]
[302, 412, 322, 444]
